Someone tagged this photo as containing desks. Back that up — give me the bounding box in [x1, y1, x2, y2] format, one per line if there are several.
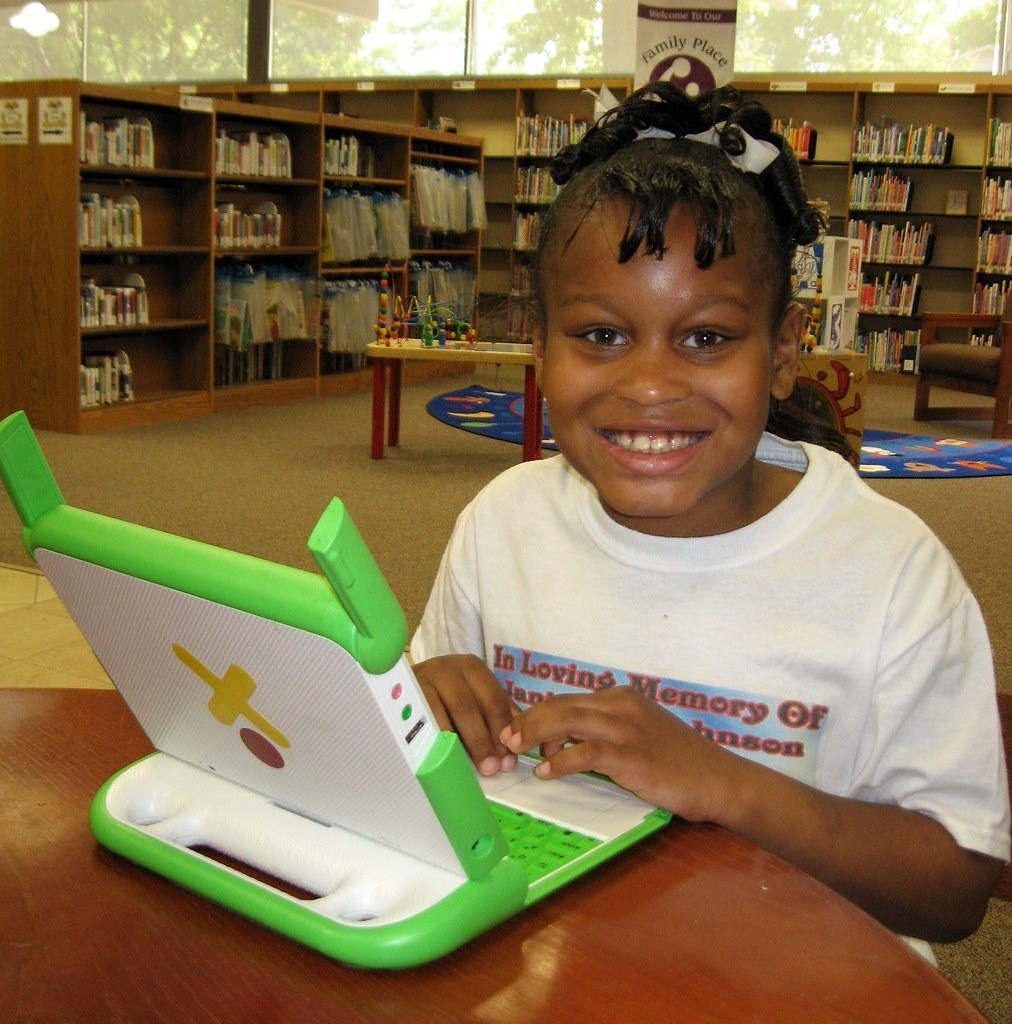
[0, 682, 984, 1024]
[366, 339, 544, 462]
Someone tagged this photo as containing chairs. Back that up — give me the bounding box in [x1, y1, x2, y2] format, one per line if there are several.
[914, 287, 1012, 439]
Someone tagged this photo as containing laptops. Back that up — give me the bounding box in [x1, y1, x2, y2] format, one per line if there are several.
[0, 411, 668, 972]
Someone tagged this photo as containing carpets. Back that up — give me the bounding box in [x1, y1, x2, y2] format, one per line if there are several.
[425, 385, 1012, 479]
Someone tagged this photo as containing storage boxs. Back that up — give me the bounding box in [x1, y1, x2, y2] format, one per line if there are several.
[785, 347, 870, 475]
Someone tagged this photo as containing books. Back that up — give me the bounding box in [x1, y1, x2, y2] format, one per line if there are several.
[214, 127, 291, 248]
[324, 135, 375, 177]
[505, 110, 595, 342]
[78, 111, 155, 408]
[771, 117, 818, 161]
[969, 117, 1012, 346]
[846, 114, 955, 373]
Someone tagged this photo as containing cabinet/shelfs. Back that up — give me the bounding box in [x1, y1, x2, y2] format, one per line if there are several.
[0, 78, 486, 439]
[121, 76, 1012, 385]
[790, 236, 864, 350]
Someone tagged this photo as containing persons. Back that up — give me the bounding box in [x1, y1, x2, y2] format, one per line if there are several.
[406, 80, 1012, 963]
